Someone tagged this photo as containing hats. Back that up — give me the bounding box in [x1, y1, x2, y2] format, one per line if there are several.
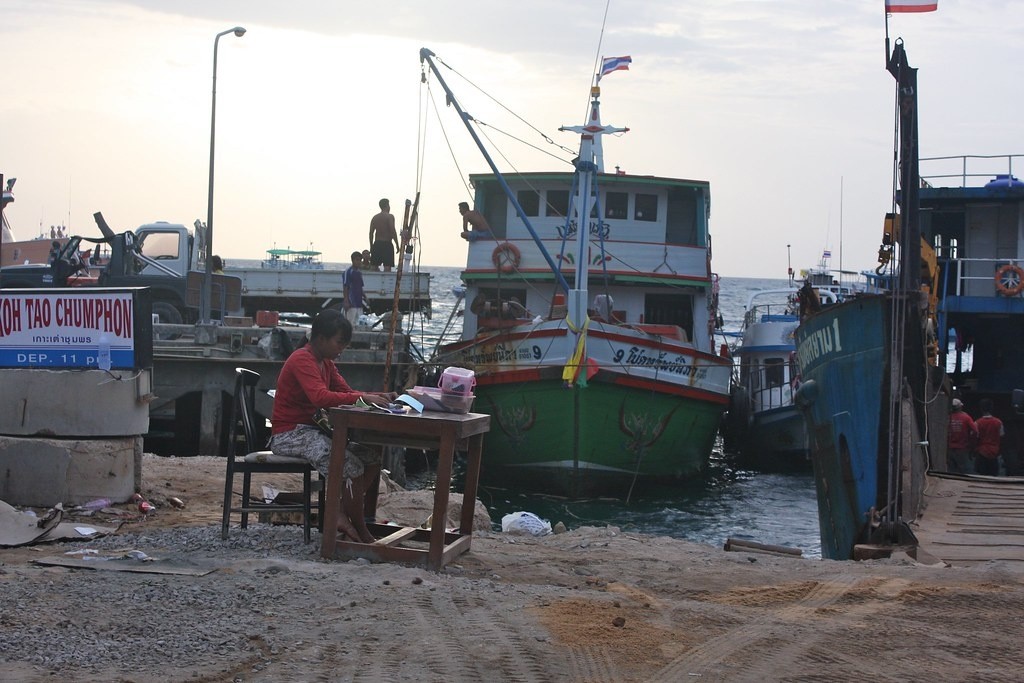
[952, 399, 961, 405]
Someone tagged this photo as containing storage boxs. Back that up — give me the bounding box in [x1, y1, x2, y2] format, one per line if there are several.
[223, 316, 253, 344]
[413, 386, 474, 396]
[406, 389, 477, 414]
[438, 367, 477, 395]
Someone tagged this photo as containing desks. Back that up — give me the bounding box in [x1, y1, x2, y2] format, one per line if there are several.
[320, 408, 491, 575]
[184, 271, 243, 324]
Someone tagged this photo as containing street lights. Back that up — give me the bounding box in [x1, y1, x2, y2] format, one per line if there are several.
[202, 26, 247, 328]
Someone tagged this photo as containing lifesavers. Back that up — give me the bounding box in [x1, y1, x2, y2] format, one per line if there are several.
[491, 242, 521, 273]
[995, 265, 1024, 295]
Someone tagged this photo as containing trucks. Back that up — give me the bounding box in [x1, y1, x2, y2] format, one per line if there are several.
[127, 222, 434, 334]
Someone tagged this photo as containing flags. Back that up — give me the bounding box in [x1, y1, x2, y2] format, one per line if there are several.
[885, 0, 938, 13]
[601, 56, 632, 75]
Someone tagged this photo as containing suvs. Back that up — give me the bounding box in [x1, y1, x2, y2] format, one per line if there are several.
[0, 233, 191, 340]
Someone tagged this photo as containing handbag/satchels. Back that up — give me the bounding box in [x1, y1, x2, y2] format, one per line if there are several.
[313, 409, 351, 440]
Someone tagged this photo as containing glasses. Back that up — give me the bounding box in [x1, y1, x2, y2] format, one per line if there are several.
[330, 333, 350, 346]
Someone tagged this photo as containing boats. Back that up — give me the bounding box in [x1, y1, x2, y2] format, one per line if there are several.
[733, 285, 849, 469]
[415, 0, 738, 499]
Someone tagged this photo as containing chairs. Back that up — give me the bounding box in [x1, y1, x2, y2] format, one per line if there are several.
[221, 369, 325, 545]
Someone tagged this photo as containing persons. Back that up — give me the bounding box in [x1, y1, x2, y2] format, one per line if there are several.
[47, 226, 100, 277]
[341, 251, 370, 329]
[458, 202, 492, 241]
[271, 309, 400, 543]
[369, 199, 399, 272]
[359, 250, 372, 271]
[212, 255, 228, 320]
[591, 287, 614, 325]
[947, 398, 1024, 476]
